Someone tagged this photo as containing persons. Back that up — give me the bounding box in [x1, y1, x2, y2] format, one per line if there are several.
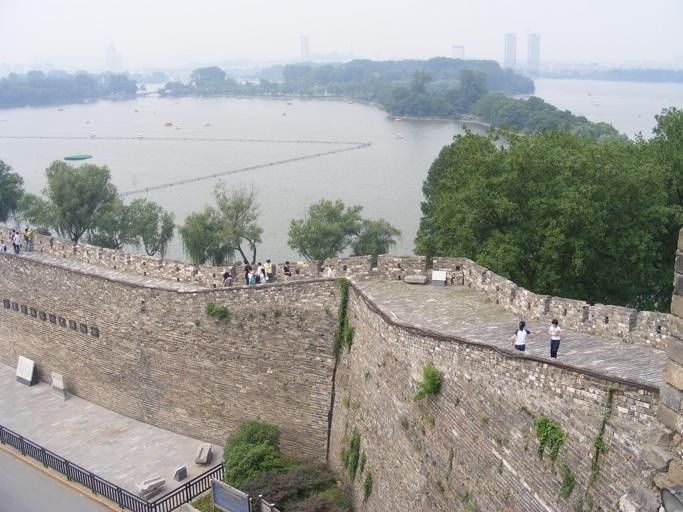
[510, 320, 541, 351]
[328, 265, 335, 278]
[282, 260, 292, 281]
[0, 228, 34, 253]
[548, 318, 561, 358]
[342, 265, 349, 276]
[243, 258, 275, 285]
[222, 272, 233, 287]
